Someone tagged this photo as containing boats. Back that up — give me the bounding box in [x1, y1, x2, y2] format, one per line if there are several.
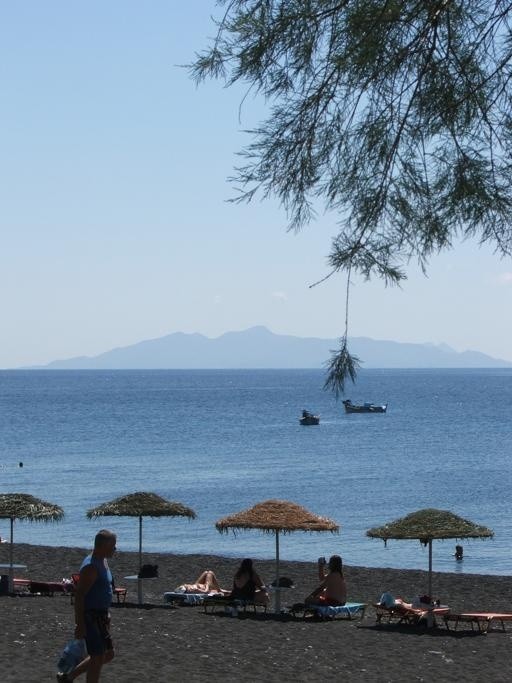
[342, 399, 387, 413]
[298, 410, 321, 424]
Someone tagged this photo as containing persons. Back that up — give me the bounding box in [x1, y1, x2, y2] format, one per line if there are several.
[453, 544, 464, 560]
[379, 591, 441, 612]
[304, 554, 347, 606]
[175, 569, 232, 594]
[230, 557, 271, 603]
[55, 528, 117, 681]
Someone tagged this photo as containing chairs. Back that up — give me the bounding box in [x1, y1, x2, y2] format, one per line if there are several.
[302, 602, 368, 621]
[165, 590, 232, 608]
[373, 602, 451, 630]
[71, 573, 81, 605]
[12, 577, 32, 596]
[113, 588, 128, 607]
[201, 596, 267, 617]
[1, 572, 10, 593]
[454, 613, 511, 631]
[235, 592, 236, 596]
[32, 580, 70, 596]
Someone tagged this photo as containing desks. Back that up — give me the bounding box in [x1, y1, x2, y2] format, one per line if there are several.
[0, 564, 28, 596]
[123, 575, 161, 608]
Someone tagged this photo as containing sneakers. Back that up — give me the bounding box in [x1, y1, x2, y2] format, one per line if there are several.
[56, 672, 73, 683]
